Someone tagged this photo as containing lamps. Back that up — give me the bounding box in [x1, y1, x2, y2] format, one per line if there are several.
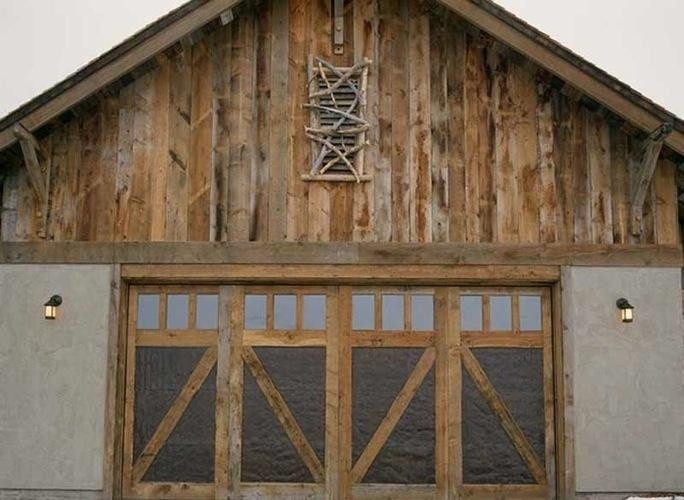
[42, 293, 62, 320]
[615, 297, 635, 323]
